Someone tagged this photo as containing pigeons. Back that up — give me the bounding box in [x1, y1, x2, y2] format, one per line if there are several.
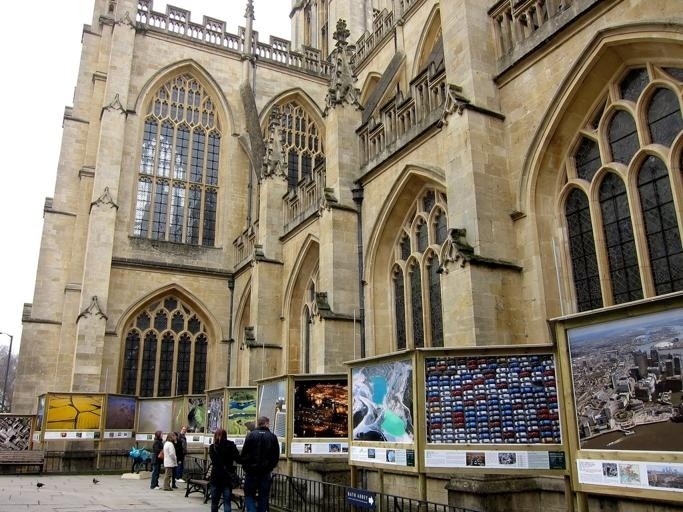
[93, 477, 100, 484]
[36, 479, 46, 489]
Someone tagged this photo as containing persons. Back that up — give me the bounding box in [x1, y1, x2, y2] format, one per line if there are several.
[174, 425, 187, 482]
[149, 430, 164, 489]
[238, 415, 280, 512]
[169, 430, 183, 489]
[206, 428, 242, 512]
[161, 432, 177, 491]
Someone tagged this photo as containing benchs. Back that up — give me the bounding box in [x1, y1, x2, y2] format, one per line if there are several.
[0, 449, 44, 476]
[217, 472, 261, 512]
[184, 463, 215, 504]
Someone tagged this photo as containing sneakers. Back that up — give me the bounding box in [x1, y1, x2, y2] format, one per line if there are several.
[163, 488, 173, 491]
[154, 487, 160, 490]
